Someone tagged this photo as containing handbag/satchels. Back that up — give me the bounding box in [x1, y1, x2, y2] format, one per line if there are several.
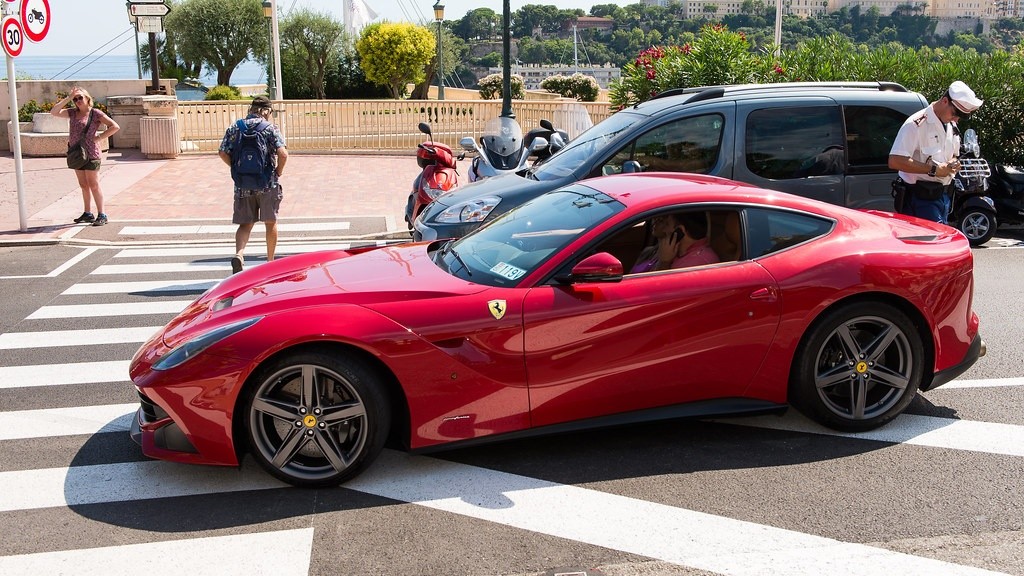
[66, 142, 90, 169]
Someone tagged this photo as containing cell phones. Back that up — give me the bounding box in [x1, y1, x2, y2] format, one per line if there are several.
[670, 228, 684, 245]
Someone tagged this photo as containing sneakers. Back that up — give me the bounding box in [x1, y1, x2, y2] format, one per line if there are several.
[92, 214, 108, 226]
[73, 211, 96, 222]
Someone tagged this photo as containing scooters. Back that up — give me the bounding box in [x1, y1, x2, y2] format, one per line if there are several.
[524, 119, 570, 166]
[404, 123, 466, 235]
[460, 117, 548, 183]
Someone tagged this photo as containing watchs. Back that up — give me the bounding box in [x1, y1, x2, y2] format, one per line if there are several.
[928, 165, 937, 177]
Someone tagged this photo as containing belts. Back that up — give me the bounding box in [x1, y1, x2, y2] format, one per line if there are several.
[895, 176, 949, 193]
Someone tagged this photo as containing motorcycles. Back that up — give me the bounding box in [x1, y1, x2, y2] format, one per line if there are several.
[985, 165, 1024, 229]
[948, 129, 998, 244]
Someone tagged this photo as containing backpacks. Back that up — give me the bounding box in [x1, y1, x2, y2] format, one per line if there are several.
[230, 119, 271, 189]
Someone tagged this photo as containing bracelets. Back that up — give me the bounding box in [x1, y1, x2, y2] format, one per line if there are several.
[98, 134, 102, 140]
[65, 98, 69, 102]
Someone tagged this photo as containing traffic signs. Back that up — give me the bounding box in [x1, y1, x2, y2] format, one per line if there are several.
[127, 0, 171, 17]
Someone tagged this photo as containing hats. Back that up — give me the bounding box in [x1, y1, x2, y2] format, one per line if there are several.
[251, 96, 272, 107]
[947, 81, 983, 118]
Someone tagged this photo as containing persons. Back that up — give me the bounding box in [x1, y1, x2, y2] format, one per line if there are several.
[51, 88, 120, 226]
[628, 209, 720, 275]
[218, 96, 288, 273]
[888, 80, 983, 228]
[614, 123, 718, 176]
[801, 144, 847, 176]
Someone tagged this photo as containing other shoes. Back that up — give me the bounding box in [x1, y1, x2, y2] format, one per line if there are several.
[231, 254, 245, 274]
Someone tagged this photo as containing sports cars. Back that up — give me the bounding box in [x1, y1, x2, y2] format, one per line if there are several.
[130, 172, 985, 487]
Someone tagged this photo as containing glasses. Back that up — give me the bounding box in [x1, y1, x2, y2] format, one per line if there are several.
[951, 104, 960, 118]
[73, 96, 83, 102]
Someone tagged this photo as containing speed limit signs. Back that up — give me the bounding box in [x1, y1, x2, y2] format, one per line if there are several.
[0, 15, 24, 58]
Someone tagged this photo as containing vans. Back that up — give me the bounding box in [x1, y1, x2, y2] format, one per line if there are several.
[409, 80, 931, 242]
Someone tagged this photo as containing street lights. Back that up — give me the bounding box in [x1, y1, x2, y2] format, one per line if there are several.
[262, 0, 277, 100]
[433, 0, 445, 100]
[125, 2, 143, 79]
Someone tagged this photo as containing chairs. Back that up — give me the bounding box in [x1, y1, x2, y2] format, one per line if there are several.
[710, 217, 742, 261]
